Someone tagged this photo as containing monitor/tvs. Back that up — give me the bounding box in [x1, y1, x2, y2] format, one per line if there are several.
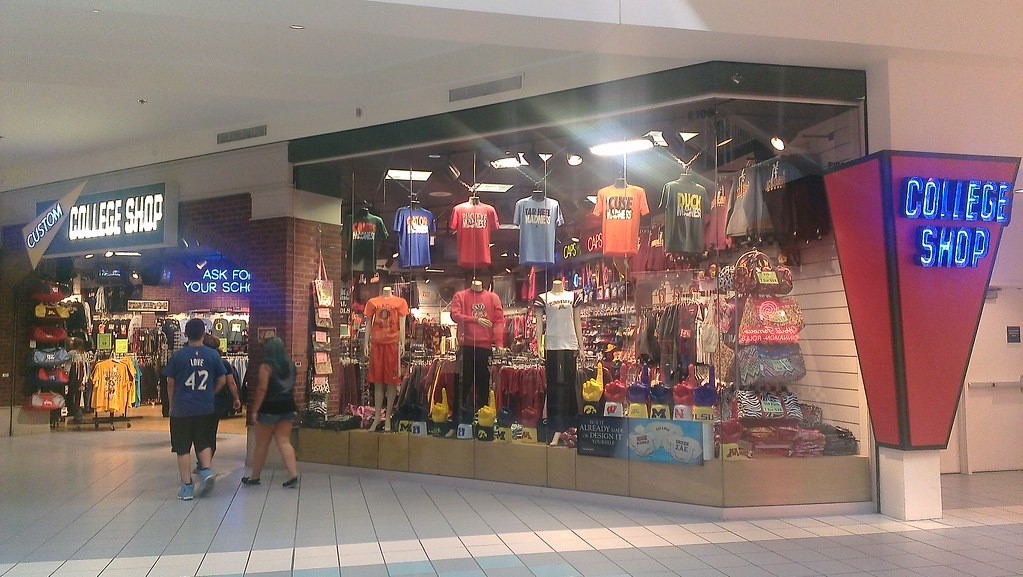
[94, 262, 128, 286]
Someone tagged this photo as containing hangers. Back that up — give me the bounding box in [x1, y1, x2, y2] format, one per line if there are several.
[71, 347, 127, 365]
[669, 289, 717, 305]
[226, 352, 250, 361]
[408, 342, 597, 370]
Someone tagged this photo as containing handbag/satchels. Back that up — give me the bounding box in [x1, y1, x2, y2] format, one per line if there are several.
[22, 276, 71, 411]
[306, 249, 336, 414]
[717, 249, 807, 426]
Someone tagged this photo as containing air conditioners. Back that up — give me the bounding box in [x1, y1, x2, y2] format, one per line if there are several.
[714, 115, 733, 147]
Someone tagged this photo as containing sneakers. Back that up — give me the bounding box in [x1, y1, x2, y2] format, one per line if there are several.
[176, 479, 197, 500]
[198, 468, 215, 497]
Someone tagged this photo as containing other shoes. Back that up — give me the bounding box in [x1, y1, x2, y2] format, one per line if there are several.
[241, 475, 262, 486]
[283, 476, 297, 487]
[193, 461, 201, 474]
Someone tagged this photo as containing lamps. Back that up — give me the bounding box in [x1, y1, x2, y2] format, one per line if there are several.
[196, 260, 208, 269]
[489, 152, 530, 169]
[770, 127, 798, 151]
[567, 151, 582, 166]
[443, 160, 460, 181]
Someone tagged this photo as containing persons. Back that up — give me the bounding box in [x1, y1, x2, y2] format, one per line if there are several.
[363, 287, 410, 432]
[66, 337, 84, 423]
[534, 280, 586, 446]
[164, 318, 240, 500]
[445, 281, 504, 438]
[242, 336, 298, 487]
